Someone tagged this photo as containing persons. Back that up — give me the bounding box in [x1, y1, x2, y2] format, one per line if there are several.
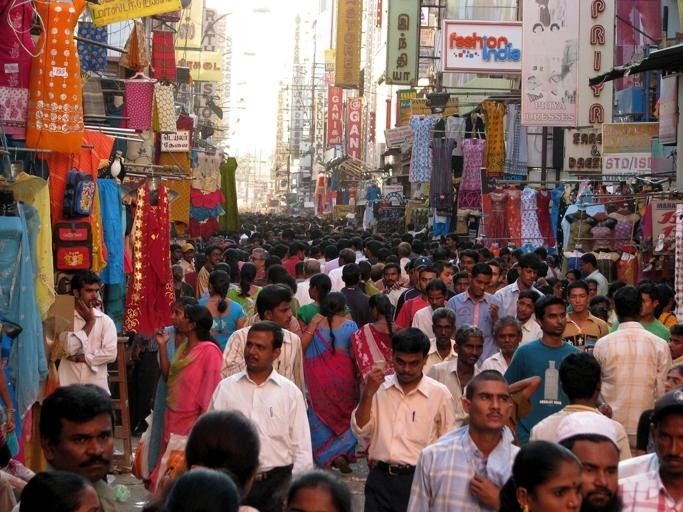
[561, 281, 609, 354]
[363, 241, 381, 265]
[164, 469, 240, 512]
[398, 243, 411, 270]
[443, 235, 459, 262]
[537, 278, 563, 300]
[179, 242, 196, 284]
[616, 179, 632, 194]
[632, 178, 645, 193]
[306, 246, 323, 261]
[497, 267, 518, 297]
[618, 386, 683, 512]
[419, 306, 459, 376]
[275, 245, 288, 262]
[0, 190, 50, 429]
[323, 246, 340, 273]
[575, 184, 595, 206]
[171, 265, 194, 300]
[494, 254, 546, 319]
[547, 24, 561, 72]
[192, 269, 246, 353]
[527, 351, 632, 462]
[529, 23, 546, 71]
[121, 173, 180, 341]
[607, 281, 628, 323]
[339, 263, 378, 328]
[563, 269, 581, 283]
[219, 156, 240, 231]
[410, 279, 449, 338]
[349, 327, 457, 511]
[355, 260, 381, 299]
[594, 286, 673, 456]
[599, 183, 612, 208]
[459, 137, 486, 210]
[286, 470, 351, 512]
[667, 323, 682, 366]
[520, 185, 544, 248]
[480, 99, 505, 178]
[537, 186, 556, 246]
[365, 181, 381, 202]
[563, 242, 588, 279]
[558, 433, 622, 511]
[507, 100, 530, 179]
[141, 410, 260, 512]
[454, 273, 472, 295]
[567, 279, 598, 313]
[58, 269, 119, 441]
[11, 384, 118, 512]
[250, 247, 270, 281]
[349, 294, 405, 470]
[412, 239, 423, 255]
[280, 243, 306, 276]
[394, 255, 433, 322]
[592, 245, 620, 283]
[221, 283, 309, 418]
[19, 470, 101, 512]
[433, 245, 456, 268]
[328, 248, 356, 292]
[27, 0, 84, 155]
[635, 363, 682, 456]
[202, 263, 242, 310]
[240, 263, 263, 313]
[296, 262, 306, 275]
[550, 182, 566, 243]
[588, 211, 618, 253]
[533, 262, 552, 289]
[383, 263, 402, 292]
[444, 114, 466, 158]
[407, 369, 522, 512]
[266, 264, 300, 317]
[265, 255, 281, 267]
[427, 325, 485, 427]
[503, 295, 583, 446]
[147, 304, 223, 498]
[397, 266, 438, 329]
[609, 282, 670, 343]
[374, 254, 408, 290]
[487, 259, 505, 293]
[189, 211, 442, 245]
[427, 136, 457, 208]
[655, 282, 680, 330]
[588, 295, 616, 328]
[223, 248, 240, 282]
[0, 0, 34, 138]
[481, 316, 523, 377]
[504, 184, 523, 247]
[446, 262, 505, 367]
[293, 258, 321, 306]
[304, 292, 360, 473]
[483, 185, 509, 248]
[460, 249, 480, 271]
[0, 357, 17, 470]
[170, 243, 181, 264]
[609, 207, 640, 250]
[296, 273, 353, 328]
[408, 113, 436, 183]
[206, 318, 316, 511]
[196, 245, 223, 301]
[616, 251, 639, 287]
[432, 260, 455, 301]
[497, 440, 584, 512]
[142, 295, 200, 488]
[581, 253, 610, 297]
[565, 210, 597, 252]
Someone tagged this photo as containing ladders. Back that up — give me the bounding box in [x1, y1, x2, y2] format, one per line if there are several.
[106, 337, 132, 468]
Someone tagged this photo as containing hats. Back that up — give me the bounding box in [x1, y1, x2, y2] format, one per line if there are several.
[182, 243, 195, 254]
[654, 390, 683, 416]
[414, 256, 433, 271]
[558, 412, 623, 453]
[591, 305, 607, 318]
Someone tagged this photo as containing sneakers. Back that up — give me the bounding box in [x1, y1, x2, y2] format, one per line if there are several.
[329, 457, 352, 477]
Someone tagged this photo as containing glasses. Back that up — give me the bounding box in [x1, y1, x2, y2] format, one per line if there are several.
[250, 256, 263, 262]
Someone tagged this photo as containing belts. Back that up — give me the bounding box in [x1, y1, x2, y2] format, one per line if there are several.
[254, 467, 283, 482]
[374, 461, 418, 478]
